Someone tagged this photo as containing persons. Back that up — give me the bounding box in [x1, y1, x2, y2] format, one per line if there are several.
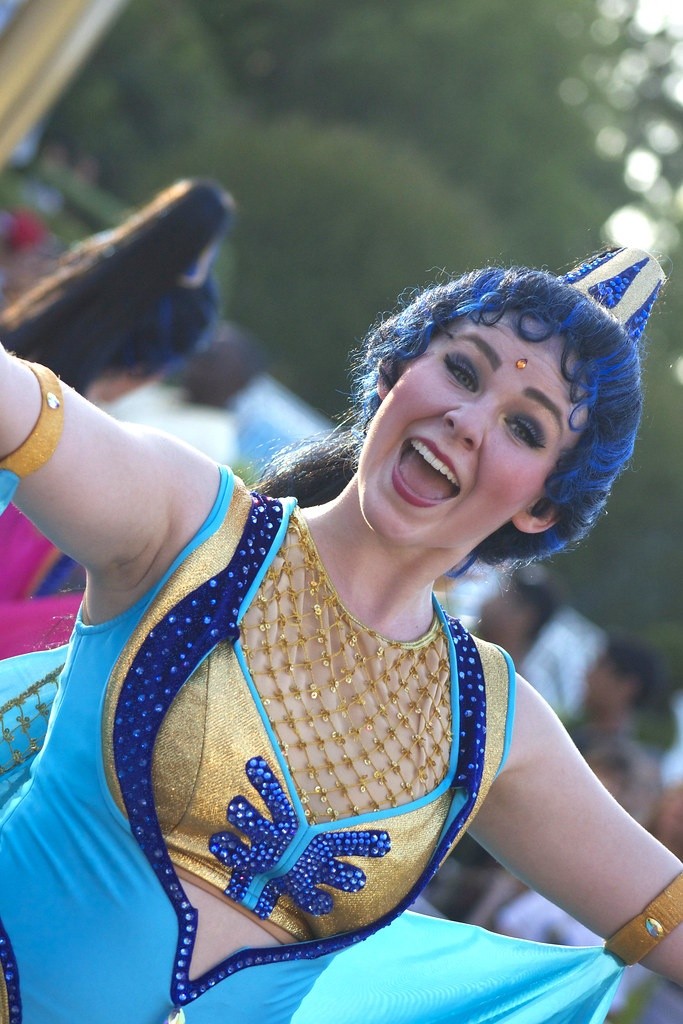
[468, 634, 661, 929]
[0, 179, 235, 657]
[0, 246, 683, 1024]
[421, 563, 562, 922]
